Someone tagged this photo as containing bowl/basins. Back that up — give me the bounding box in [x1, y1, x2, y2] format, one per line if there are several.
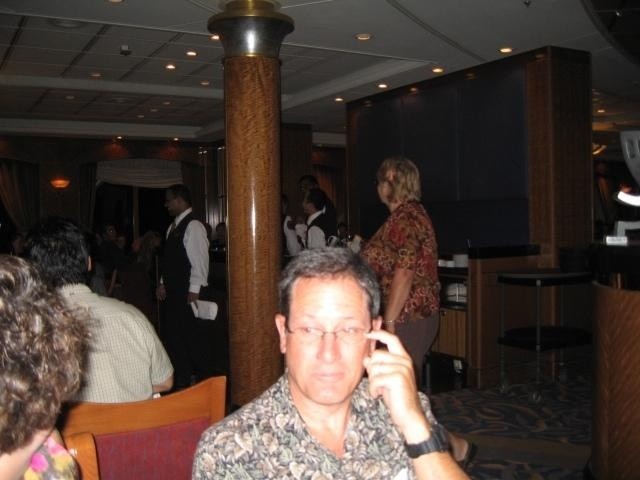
[443, 283, 468, 305]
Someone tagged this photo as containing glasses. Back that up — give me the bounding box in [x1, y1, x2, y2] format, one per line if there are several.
[285, 327, 367, 345]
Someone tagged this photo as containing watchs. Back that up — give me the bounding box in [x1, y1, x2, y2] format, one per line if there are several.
[403, 421, 450, 459]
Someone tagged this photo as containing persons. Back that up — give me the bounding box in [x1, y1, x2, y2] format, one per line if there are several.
[85, 223, 160, 331]
[0, 254, 85, 480]
[358, 155, 478, 472]
[160, 186, 211, 396]
[203, 224, 226, 265]
[11, 216, 175, 403]
[280, 174, 341, 256]
[189, 246, 471, 480]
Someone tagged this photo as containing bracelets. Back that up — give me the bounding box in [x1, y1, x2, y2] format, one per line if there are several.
[383, 320, 396, 324]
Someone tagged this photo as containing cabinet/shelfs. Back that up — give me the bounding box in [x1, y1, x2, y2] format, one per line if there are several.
[429, 307, 468, 363]
[497, 267, 592, 404]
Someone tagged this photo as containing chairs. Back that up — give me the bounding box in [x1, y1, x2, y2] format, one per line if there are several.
[46, 376, 228, 480]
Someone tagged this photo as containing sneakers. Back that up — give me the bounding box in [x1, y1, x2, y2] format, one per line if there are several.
[456, 441, 477, 470]
